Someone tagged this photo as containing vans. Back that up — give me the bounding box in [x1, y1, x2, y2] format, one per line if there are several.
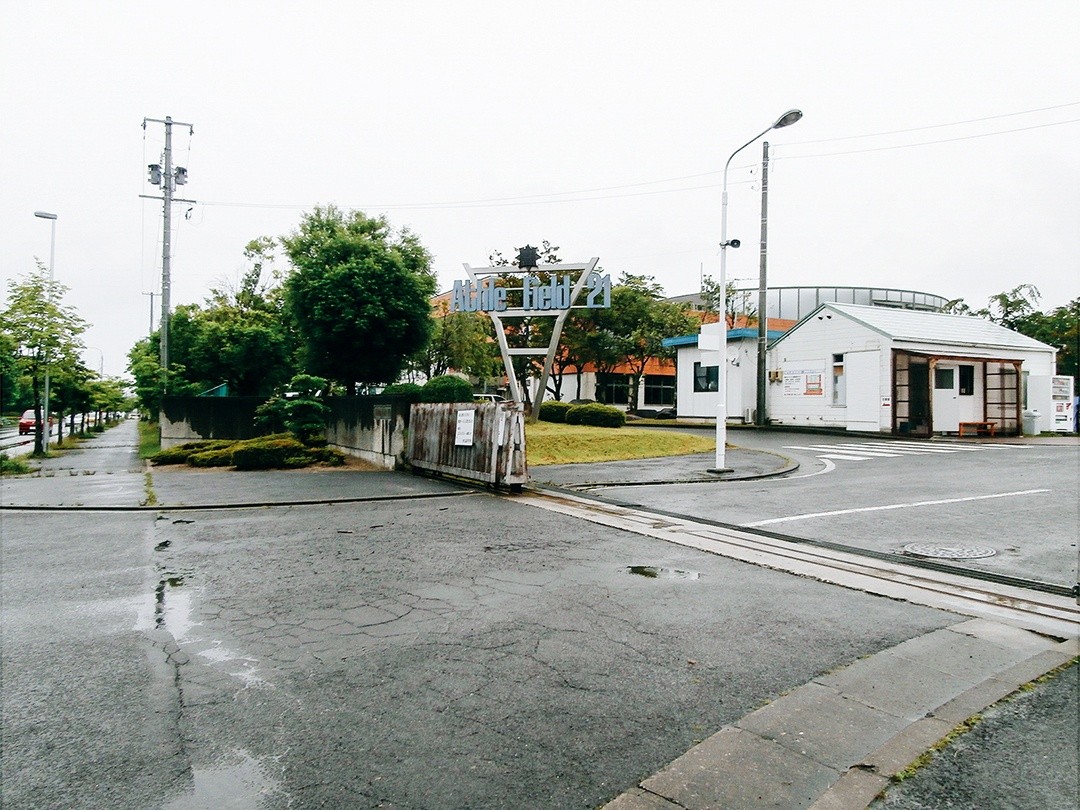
[473, 393, 525, 424]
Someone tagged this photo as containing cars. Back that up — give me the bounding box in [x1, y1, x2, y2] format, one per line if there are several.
[18, 409, 53, 435]
[65, 410, 126, 427]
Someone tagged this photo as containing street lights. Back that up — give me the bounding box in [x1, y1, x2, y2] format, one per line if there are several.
[34, 210, 58, 453]
[708, 108, 804, 473]
